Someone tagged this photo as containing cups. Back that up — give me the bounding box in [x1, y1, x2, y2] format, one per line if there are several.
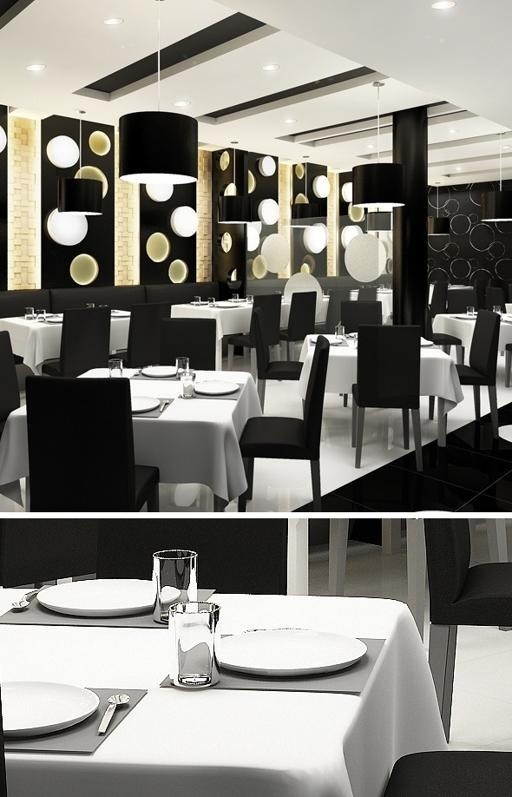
[180, 369, 195, 399]
[175, 356, 190, 380]
[164, 600, 223, 690]
[456, 302, 512, 322]
[23, 305, 63, 323]
[108, 358, 123, 378]
[190, 292, 254, 309]
[357, 281, 394, 294]
[86, 302, 108, 309]
[150, 547, 199, 625]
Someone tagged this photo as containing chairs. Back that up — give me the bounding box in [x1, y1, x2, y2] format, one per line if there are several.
[1, 277, 509, 509]
[378, 753, 510, 796]
[98, 514, 289, 595]
[421, 515, 511, 748]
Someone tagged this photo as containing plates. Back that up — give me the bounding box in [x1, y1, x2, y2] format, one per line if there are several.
[420, 339, 434, 347]
[131, 395, 160, 413]
[0, 680, 101, 743]
[110, 310, 131, 317]
[35, 577, 154, 618]
[141, 365, 176, 378]
[212, 626, 370, 678]
[193, 380, 240, 395]
[309, 324, 358, 350]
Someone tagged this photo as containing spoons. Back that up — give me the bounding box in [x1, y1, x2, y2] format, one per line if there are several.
[10, 588, 39, 610]
[158, 399, 170, 412]
[133, 369, 141, 377]
[97, 692, 131, 737]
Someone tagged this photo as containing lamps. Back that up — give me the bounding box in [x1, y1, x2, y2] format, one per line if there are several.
[427, 180, 448, 237]
[56, 111, 101, 220]
[352, 80, 406, 213]
[478, 121, 510, 227]
[119, 2, 199, 195]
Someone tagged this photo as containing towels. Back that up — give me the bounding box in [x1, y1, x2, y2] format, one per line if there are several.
[164, 632, 385, 698]
[1, 684, 153, 758]
[1, 588, 215, 628]
